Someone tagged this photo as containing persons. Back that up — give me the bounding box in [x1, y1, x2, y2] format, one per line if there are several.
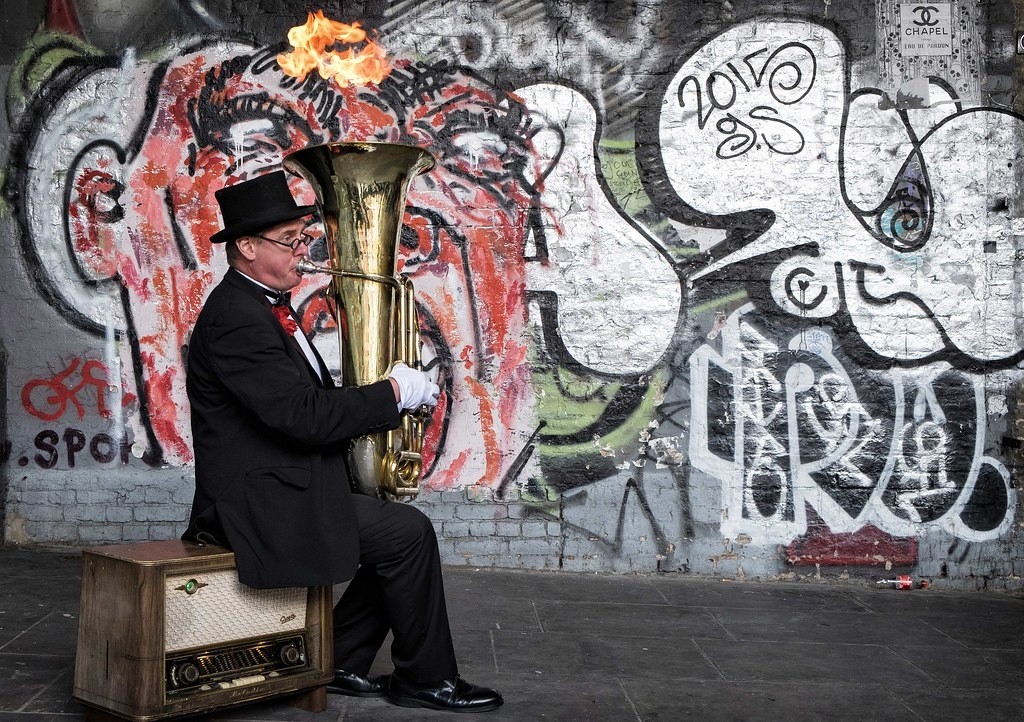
[178, 171, 505, 712]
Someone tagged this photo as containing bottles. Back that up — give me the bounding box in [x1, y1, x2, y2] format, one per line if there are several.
[870, 575, 928, 590]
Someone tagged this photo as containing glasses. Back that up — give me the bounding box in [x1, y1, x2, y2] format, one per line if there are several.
[252, 231, 314, 250]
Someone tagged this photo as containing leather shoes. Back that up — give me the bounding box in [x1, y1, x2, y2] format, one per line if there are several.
[389, 672, 504, 713]
[326, 667, 391, 698]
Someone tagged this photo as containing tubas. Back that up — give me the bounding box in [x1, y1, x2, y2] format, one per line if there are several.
[281, 141, 440, 506]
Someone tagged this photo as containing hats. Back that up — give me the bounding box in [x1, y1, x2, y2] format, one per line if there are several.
[210, 171, 317, 243]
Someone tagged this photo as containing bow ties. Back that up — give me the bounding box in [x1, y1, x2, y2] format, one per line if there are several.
[259, 286, 291, 306]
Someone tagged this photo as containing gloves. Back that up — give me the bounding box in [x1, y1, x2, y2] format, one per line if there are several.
[379, 362, 440, 413]
